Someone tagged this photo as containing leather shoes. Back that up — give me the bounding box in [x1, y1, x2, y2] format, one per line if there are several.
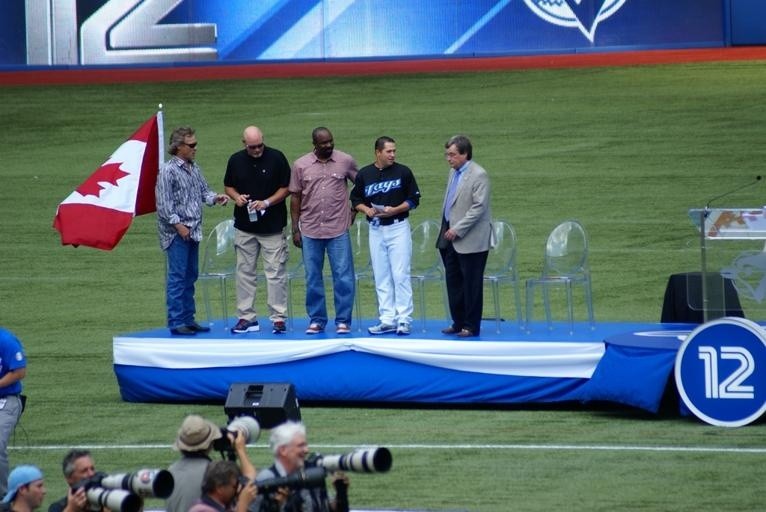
[187, 324, 210, 332]
[456, 330, 473, 337]
[336, 323, 351, 334]
[170, 326, 197, 336]
[441, 328, 456, 334]
[305, 324, 324, 334]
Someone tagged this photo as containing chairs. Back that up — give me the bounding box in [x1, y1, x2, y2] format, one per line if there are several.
[526, 220, 596, 337]
[482, 223, 523, 334]
[323, 221, 374, 330]
[411, 220, 450, 332]
[198, 220, 239, 328]
[256, 220, 306, 331]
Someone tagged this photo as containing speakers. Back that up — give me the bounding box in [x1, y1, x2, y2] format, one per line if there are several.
[223, 381, 302, 430]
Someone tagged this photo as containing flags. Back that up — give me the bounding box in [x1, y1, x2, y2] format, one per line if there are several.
[51, 103, 165, 252]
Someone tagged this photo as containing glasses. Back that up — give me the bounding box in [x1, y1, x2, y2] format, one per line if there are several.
[244, 142, 263, 149]
[183, 143, 197, 148]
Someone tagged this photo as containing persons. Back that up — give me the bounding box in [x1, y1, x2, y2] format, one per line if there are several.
[165, 415, 256, 512]
[71, 448, 113, 512]
[223, 125, 291, 334]
[187, 460, 259, 512]
[435, 134, 497, 337]
[0, 465, 46, 512]
[1, 328, 26, 500]
[247, 421, 351, 511]
[288, 126, 361, 334]
[349, 136, 422, 337]
[154, 126, 229, 336]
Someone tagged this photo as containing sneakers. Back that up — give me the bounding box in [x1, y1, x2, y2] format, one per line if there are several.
[397, 321, 410, 335]
[272, 321, 287, 334]
[231, 319, 259, 333]
[367, 323, 397, 334]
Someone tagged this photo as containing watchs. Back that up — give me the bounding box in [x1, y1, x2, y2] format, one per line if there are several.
[292, 228, 300, 235]
[263, 200, 270, 207]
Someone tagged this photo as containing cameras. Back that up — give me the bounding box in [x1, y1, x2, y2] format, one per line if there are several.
[253, 468, 328, 494]
[71, 477, 144, 512]
[89, 468, 175, 499]
[303, 445, 393, 479]
[211, 414, 261, 452]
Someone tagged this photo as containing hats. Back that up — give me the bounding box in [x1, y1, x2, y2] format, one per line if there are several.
[176, 415, 222, 452]
[1, 465, 44, 503]
